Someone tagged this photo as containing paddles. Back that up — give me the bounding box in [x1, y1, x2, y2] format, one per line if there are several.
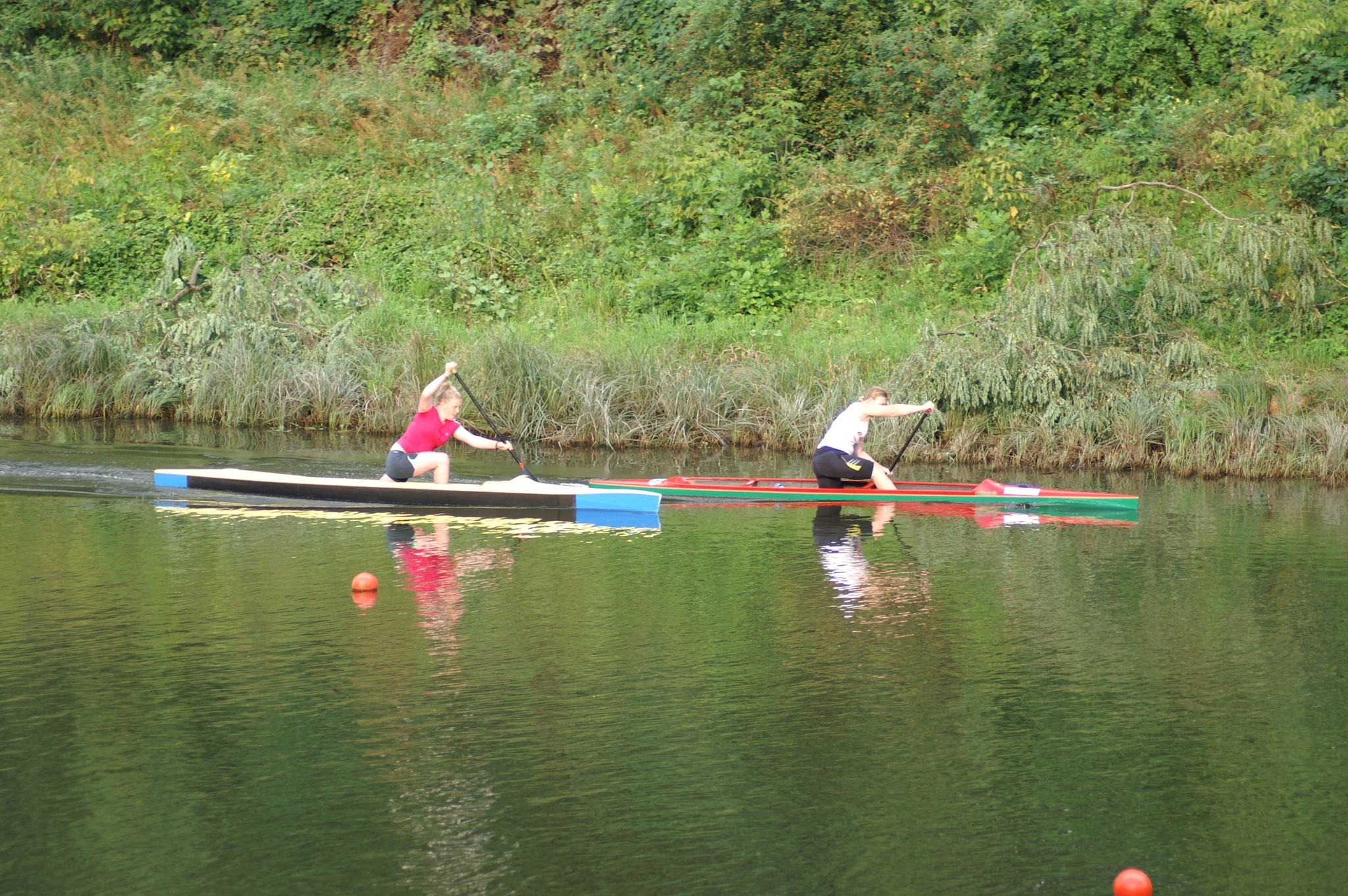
[873, 408, 932, 487]
[449, 367, 538, 482]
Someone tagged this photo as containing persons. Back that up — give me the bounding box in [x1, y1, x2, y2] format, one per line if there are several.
[379, 362, 513, 484]
[812, 386, 935, 490]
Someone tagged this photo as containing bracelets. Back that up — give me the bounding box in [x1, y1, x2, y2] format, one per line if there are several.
[495, 441, 499, 451]
[443, 373, 450, 381]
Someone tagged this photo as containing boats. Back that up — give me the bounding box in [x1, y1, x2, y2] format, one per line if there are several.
[153, 467, 662, 513]
[586, 477, 1140, 509]
